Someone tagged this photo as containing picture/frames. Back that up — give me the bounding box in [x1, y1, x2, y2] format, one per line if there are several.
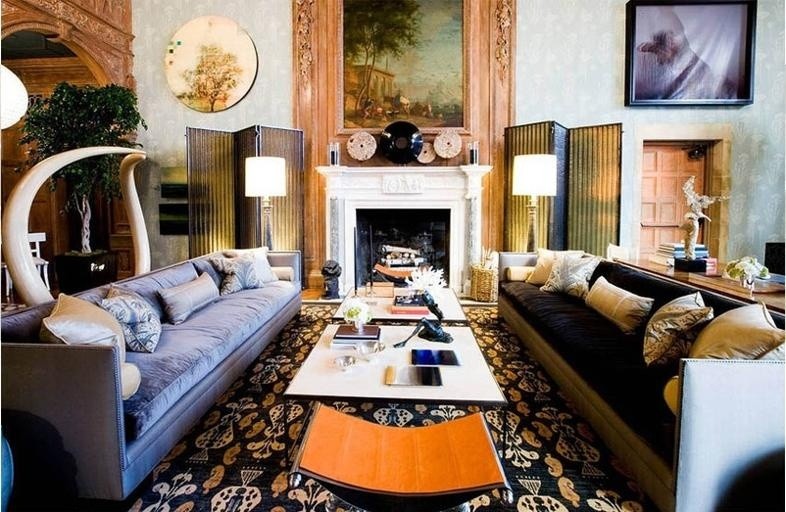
[625, 0, 758, 107]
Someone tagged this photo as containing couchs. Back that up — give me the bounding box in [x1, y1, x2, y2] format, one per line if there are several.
[494, 249, 785, 512]
[1, 246, 301, 502]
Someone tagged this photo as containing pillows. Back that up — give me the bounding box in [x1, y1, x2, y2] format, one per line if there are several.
[221, 246, 279, 283]
[208, 252, 265, 296]
[584, 275, 655, 336]
[95, 282, 162, 353]
[39, 292, 141, 401]
[641, 292, 782, 414]
[539, 252, 602, 300]
[506, 266, 535, 281]
[525, 248, 584, 287]
[155, 271, 220, 326]
[271, 267, 294, 281]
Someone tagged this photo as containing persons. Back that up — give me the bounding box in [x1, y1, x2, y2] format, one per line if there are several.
[683, 195, 711, 260]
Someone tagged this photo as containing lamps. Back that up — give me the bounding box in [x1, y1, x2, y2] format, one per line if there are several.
[511, 154, 558, 253]
[243, 156, 287, 250]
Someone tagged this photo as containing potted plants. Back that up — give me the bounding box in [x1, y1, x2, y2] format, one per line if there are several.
[15, 80, 149, 295]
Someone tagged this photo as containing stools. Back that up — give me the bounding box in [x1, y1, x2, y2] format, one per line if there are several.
[287, 399, 513, 512]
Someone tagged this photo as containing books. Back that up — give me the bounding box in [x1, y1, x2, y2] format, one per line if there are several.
[649, 241, 717, 266]
[387, 295, 429, 315]
[385, 366, 442, 387]
[412, 350, 459, 366]
[333, 325, 380, 344]
